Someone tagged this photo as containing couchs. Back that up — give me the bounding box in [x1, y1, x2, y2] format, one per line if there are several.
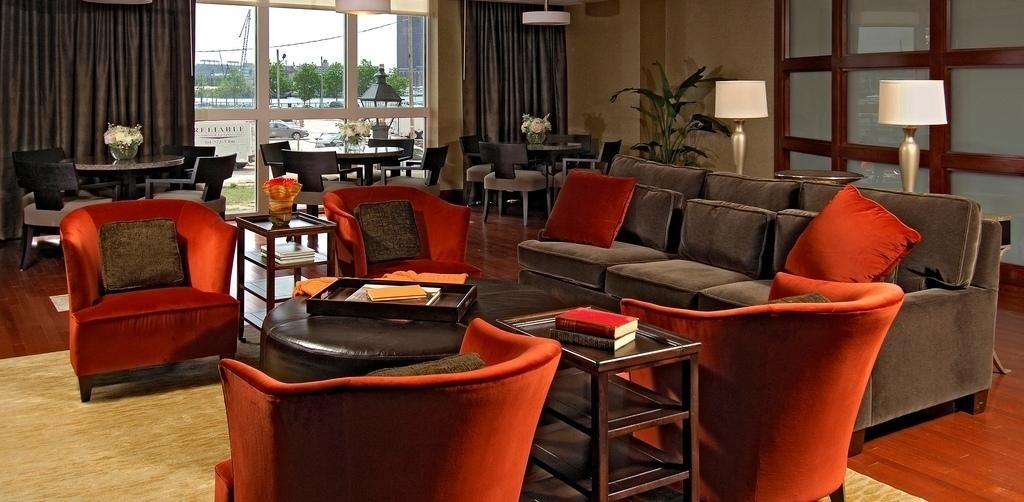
[518, 220, 1003, 459]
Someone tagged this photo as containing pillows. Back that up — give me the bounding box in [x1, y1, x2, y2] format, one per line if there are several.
[96, 218, 184, 291]
[364, 352, 484, 375]
[799, 182, 982, 289]
[767, 292, 833, 303]
[542, 154, 800, 280]
[354, 200, 421, 263]
[772, 209, 898, 283]
[785, 183, 921, 283]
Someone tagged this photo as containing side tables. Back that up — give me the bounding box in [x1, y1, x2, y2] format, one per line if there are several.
[235, 211, 337, 344]
[497, 305, 702, 502]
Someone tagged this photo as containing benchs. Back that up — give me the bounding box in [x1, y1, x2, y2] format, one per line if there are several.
[259, 277, 618, 383]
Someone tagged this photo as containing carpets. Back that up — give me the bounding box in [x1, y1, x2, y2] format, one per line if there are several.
[0, 322, 926, 502]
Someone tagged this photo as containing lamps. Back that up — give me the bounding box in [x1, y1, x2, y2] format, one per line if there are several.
[715, 80, 768, 174]
[879, 79, 949, 194]
[522, 0, 571, 26]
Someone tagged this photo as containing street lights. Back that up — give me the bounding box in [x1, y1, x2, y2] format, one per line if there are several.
[319, 56, 327, 108]
[275, 49, 286, 107]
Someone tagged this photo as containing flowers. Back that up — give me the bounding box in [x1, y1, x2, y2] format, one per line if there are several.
[520, 112, 552, 133]
[337, 123, 371, 138]
[104, 122, 144, 146]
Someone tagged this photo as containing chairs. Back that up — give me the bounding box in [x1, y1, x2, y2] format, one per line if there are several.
[59, 200, 240, 402]
[11, 148, 237, 273]
[620, 272, 905, 502]
[322, 186, 481, 280]
[458, 135, 622, 226]
[214, 318, 562, 502]
[261, 140, 450, 253]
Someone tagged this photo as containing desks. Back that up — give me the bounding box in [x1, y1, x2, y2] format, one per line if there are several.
[775, 170, 863, 185]
[982, 211, 1014, 374]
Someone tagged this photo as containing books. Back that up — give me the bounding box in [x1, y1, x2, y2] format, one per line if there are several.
[260, 241, 316, 265]
[344, 283, 442, 305]
[365, 284, 427, 302]
[554, 306, 640, 339]
[548, 327, 637, 352]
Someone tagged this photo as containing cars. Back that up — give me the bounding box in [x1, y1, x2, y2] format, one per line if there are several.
[329, 101, 343, 108]
[314, 131, 343, 148]
[268, 120, 309, 141]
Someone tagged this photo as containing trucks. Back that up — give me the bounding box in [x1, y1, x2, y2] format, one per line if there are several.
[194, 120, 255, 169]
